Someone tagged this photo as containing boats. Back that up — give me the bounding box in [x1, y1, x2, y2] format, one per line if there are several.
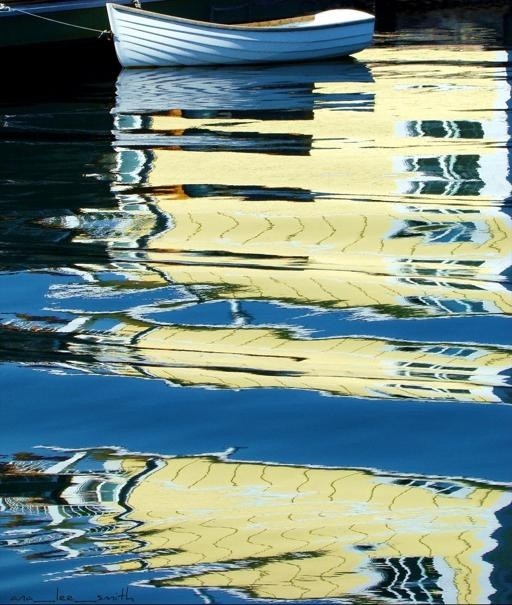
[103, 1, 378, 67]
